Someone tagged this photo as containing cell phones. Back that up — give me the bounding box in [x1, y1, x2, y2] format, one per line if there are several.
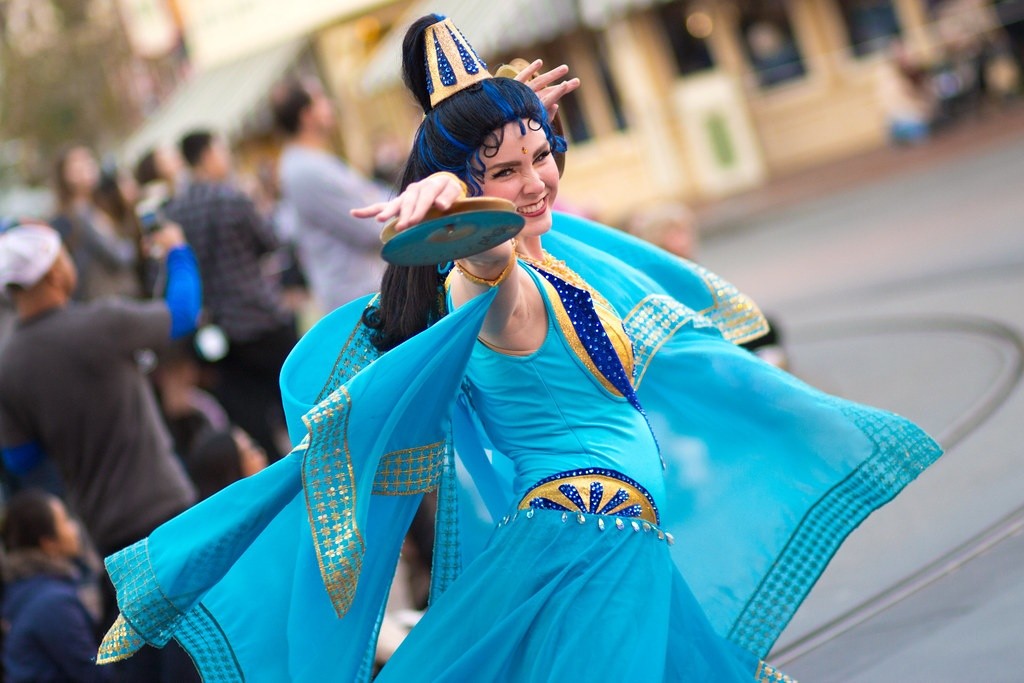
[137, 211, 160, 234]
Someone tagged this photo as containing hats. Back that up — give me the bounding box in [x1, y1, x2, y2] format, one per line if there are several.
[0, 225, 61, 295]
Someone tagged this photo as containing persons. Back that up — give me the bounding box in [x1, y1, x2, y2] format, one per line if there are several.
[95, 14, 943, 683]
[273, 75, 398, 314]
[0, 491, 135, 683]
[0, 200, 202, 545]
[44, 131, 407, 474]
